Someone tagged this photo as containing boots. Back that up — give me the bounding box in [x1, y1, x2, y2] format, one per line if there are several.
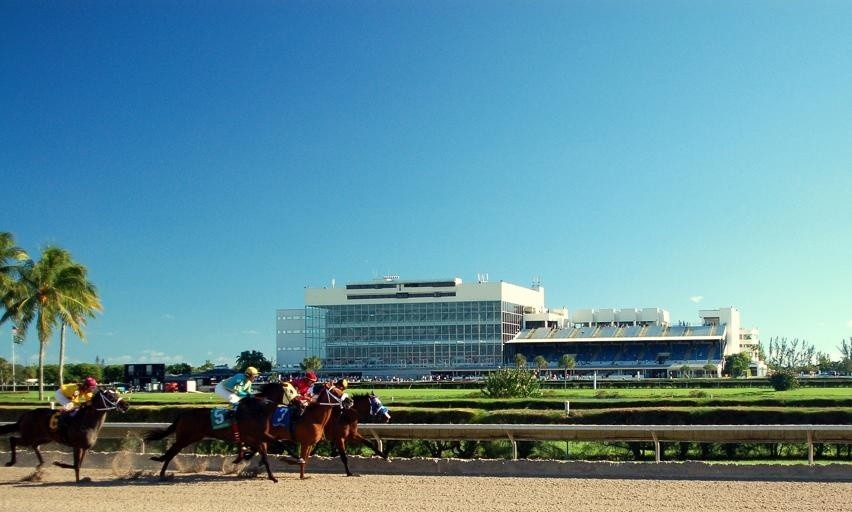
[289, 408, 305, 422]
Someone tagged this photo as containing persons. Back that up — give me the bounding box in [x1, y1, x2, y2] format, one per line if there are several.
[214, 366, 260, 410]
[54, 377, 98, 432]
[288, 370, 318, 405]
[335, 379, 348, 391]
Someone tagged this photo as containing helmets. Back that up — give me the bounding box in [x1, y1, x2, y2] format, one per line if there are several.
[84, 378, 97, 389]
[245, 366, 259, 378]
[338, 379, 347, 388]
[305, 371, 318, 382]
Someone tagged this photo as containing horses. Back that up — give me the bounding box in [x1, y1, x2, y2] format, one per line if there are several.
[233, 380, 354, 479]
[245, 379, 391, 480]
[143, 378, 307, 483]
[0, 386, 130, 483]
